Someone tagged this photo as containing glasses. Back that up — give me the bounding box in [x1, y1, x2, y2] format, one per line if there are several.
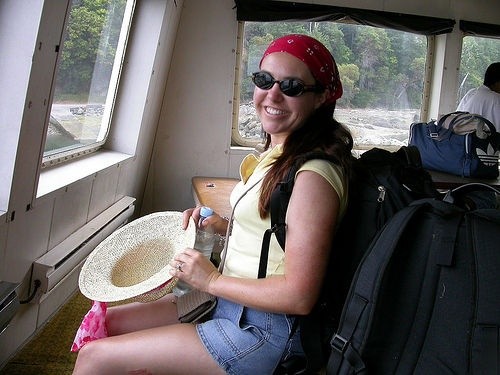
[251, 72, 318, 96]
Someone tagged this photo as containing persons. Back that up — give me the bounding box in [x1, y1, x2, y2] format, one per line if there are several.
[72, 35, 353, 375]
[457, 61, 500, 132]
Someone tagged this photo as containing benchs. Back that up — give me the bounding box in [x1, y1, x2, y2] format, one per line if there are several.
[191, 176, 241, 220]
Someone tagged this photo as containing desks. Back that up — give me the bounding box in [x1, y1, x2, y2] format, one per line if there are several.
[427, 168, 500, 192]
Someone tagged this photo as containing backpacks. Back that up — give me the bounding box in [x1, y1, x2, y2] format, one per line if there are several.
[257, 146, 442, 365]
[325, 182, 500, 375]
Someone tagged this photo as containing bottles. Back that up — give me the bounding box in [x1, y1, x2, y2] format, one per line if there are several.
[173, 206, 215, 297]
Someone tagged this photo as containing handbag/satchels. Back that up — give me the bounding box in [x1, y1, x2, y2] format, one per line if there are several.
[176, 288, 216, 323]
[408, 112, 500, 180]
[437, 112, 491, 139]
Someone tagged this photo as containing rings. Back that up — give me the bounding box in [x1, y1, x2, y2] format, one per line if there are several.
[177, 263, 182, 268]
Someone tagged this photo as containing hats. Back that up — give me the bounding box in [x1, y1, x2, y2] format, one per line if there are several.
[69, 211, 196, 353]
